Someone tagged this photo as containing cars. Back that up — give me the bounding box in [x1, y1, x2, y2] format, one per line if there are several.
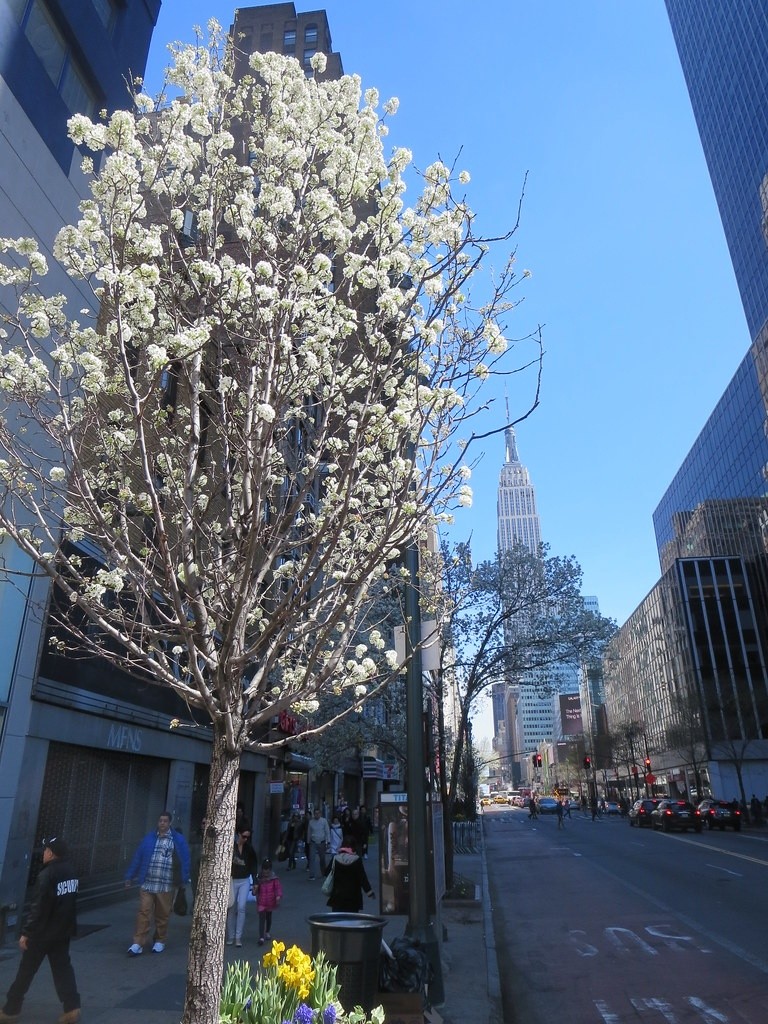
[483, 791, 580, 815]
[599, 801, 621, 814]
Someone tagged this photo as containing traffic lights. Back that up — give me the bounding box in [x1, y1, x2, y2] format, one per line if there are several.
[585, 757, 590, 769]
[537, 755, 542, 767]
[646, 759, 651, 770]
[532, 755, 537, 767]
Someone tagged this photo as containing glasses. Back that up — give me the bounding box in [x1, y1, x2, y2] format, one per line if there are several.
[240, 833, 250, 840]
[294, 815, 299, 817]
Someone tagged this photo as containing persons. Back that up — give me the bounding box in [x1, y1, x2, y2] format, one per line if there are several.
[481, 794, 638, 829]
[0, 837, 82, 1024]
[733, 794, 768, 823]
[125, 812, 191, 955]
[226, 793, 375, 946]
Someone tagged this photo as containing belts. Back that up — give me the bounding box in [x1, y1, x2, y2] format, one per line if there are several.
[311, 840, 325, 843]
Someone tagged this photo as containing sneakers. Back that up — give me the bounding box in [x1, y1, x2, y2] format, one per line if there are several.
[152, 942, 165, 954]
[127, 944, 143, 954]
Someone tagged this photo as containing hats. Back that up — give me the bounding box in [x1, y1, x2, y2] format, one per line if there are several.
[42, 835, 68, 857]
[262, 857, 272, 870]
[399, 805, 408, 816]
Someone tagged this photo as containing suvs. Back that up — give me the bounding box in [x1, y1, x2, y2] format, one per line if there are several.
[628, 798, 663, 828]
[650, 800, 702, 833]
[698, 798, 741, 831]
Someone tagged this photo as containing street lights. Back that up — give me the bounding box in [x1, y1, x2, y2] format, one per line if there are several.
[371, 260, 445, 1012]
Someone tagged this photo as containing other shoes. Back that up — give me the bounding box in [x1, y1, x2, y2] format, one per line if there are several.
[364, 853, 369, 859]
[0, 1010, 20, 1024]
[266, 934, 270, 940]
[309, 877, 315, 881]
[258, 938, 264, 944]
[59, 1008, 81, 1024]
[236, 938, 242, 946]
[226, 938, 233, 945]
[190, 907, 193, 913]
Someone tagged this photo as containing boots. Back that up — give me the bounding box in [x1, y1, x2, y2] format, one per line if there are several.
[287, 859, 296, 871]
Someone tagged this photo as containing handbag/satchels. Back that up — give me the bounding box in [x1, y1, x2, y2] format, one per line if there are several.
[237, 874, 256, 903]
[321, 856, 336, 896]
[276, 845, 288, 862]
[227, 876, 234, 909]
[173, 887, 187, 916]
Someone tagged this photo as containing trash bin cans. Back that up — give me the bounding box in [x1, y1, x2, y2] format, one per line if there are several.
[305, 913, 390, 1024]
